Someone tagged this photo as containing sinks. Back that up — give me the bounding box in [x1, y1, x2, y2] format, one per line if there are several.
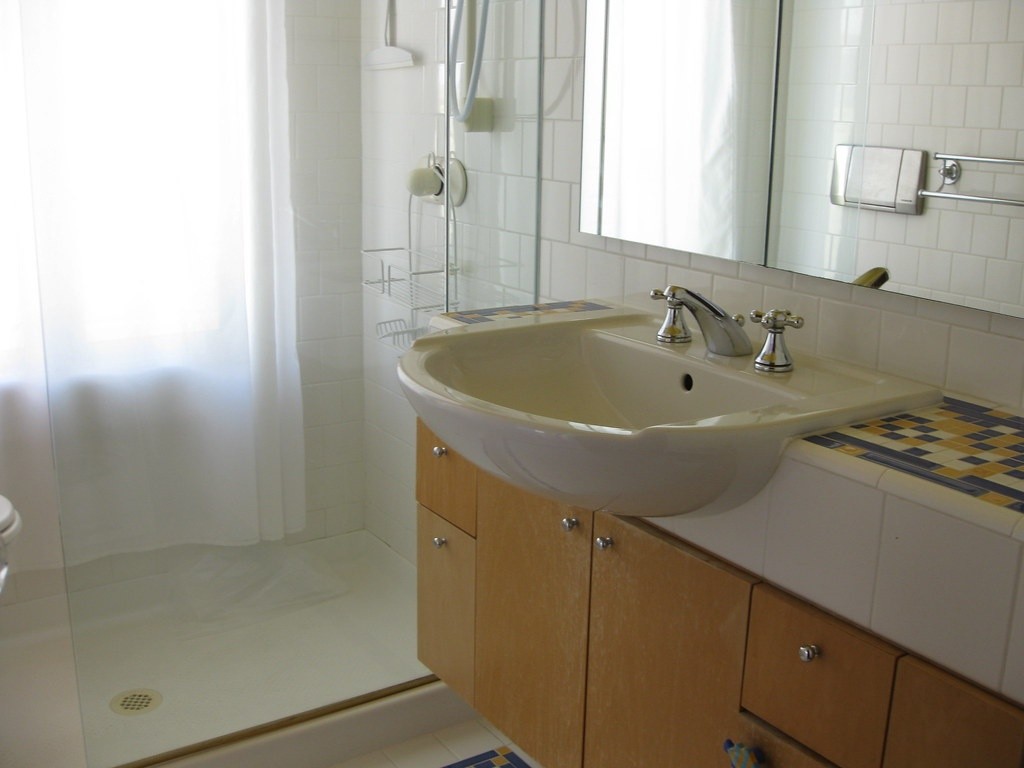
[395, 305, 944, 518]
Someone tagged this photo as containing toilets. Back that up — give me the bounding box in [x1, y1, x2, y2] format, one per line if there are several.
[0, 494, 24, 570]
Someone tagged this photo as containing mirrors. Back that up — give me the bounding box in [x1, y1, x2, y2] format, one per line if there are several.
[578, 0, 1024, 322]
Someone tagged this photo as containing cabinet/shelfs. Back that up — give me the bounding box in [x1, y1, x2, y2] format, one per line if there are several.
[413, 417, 1024, 768]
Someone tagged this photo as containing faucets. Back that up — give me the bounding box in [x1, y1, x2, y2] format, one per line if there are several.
[663, 284, 754, 358]
[850, 266, 891, 290]
